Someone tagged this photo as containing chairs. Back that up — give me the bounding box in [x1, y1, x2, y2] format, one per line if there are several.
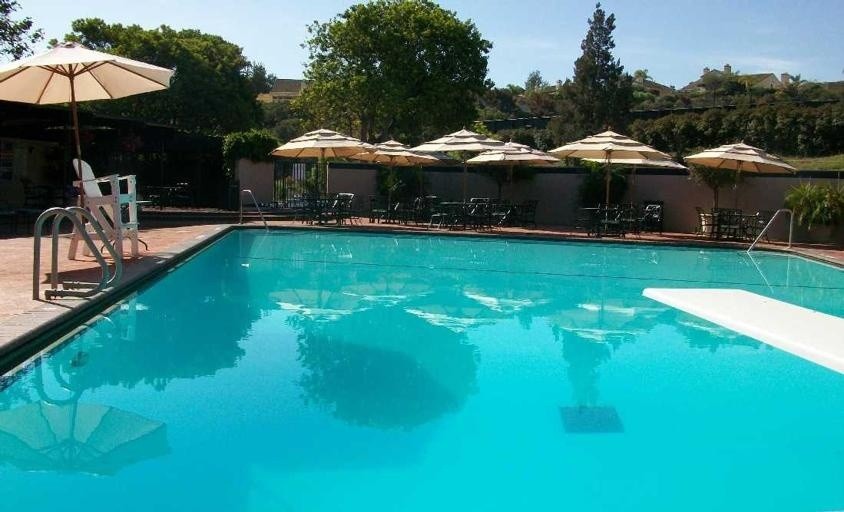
[269, 192, 357, 230]
[578, 199, 666, 243]
[0, 178, 195, 240]
[693, 203, 768, 244]
[365, 192, 540, 238]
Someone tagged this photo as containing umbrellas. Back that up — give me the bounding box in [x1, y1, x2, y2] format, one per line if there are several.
[0, 40, 174, 231]
[0, 330, 174, 480]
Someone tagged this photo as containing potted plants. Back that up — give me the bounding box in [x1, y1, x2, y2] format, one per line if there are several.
[782, 176, 844, 242]
